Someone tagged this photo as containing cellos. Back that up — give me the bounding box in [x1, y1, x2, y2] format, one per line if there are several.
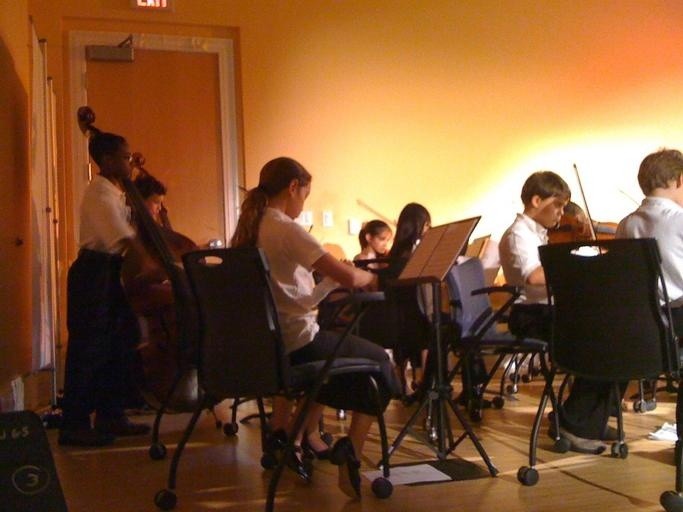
[132, 150, 171, 231]
[77, 105, 207, 414]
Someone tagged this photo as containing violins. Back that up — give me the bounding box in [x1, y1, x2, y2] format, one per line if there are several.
[547, 199, 619, 245]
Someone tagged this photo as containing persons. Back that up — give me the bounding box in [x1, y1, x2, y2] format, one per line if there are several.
[232, 157, 394, 503]
[128, 174, 168, 225]
[262, 390, 334, 463]
[495, 168, 615, 455]
[352, 220, 393, 272]
[614, 150, 683, 337]
[396, 201, 435, 406]
[57, 131, 146, 446]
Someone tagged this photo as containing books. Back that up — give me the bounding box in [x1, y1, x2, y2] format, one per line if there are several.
[462, 235, 501, 284]
[399, 217, 477, 279]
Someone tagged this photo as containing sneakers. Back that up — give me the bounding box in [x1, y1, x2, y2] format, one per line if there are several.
[548, 424, 605, 454]
[94, 415, 151, 436]
[58, 428, 115, 448]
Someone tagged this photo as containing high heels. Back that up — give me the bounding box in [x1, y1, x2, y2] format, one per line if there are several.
[330, 437, 361, 500]
[268, 430, 311, 480]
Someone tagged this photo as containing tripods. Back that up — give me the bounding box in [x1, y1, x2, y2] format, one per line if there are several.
[381, 279, 499, 476]
